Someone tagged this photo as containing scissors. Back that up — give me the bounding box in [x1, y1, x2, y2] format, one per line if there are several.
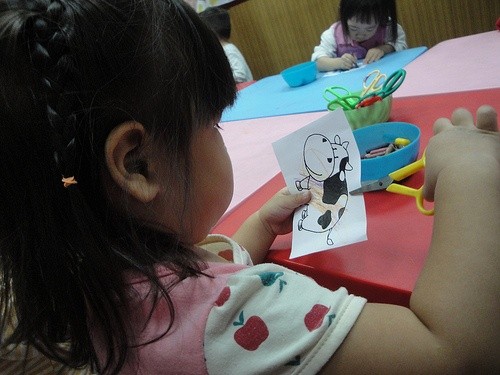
[349, 146, 435, 216]
[321, 69, 406, 112]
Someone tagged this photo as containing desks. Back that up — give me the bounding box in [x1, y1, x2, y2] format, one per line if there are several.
[203, 29, 500, 308]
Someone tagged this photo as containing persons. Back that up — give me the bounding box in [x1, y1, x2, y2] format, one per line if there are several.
[198, 7, 254, 83]
[311, 0, 409, 71]
[0, 0, 500, 375]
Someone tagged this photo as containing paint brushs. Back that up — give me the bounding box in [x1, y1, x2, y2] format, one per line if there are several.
[353, 61, 359, 67]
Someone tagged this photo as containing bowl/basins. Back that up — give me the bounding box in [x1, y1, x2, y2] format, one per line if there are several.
[328, 88, 392, 129]
[353, 122, 421, 180]
[281, 61, 319, 88]
